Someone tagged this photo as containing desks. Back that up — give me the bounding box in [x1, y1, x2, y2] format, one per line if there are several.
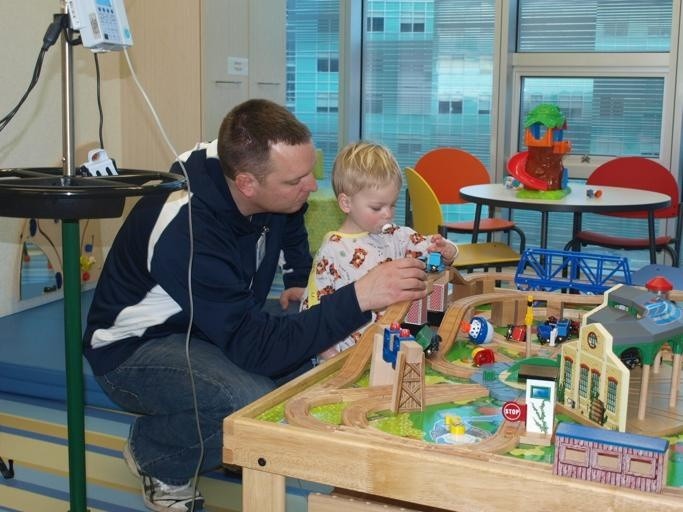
[223, 287, 683, 511]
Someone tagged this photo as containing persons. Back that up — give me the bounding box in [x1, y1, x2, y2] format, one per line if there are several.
[82, 99, 427, 512]
[299, 142, 460, 366]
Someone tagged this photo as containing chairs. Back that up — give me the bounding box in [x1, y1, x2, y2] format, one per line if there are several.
[562, 155, 682, 293]
[405, 167, 523, 286]
[415, 149, 526, 256]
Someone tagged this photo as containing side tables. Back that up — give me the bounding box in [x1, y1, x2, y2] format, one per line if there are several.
[457, 181, 672, 293]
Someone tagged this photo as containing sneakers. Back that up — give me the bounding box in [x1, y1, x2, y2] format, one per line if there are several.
[121, 429, 205, 512]
